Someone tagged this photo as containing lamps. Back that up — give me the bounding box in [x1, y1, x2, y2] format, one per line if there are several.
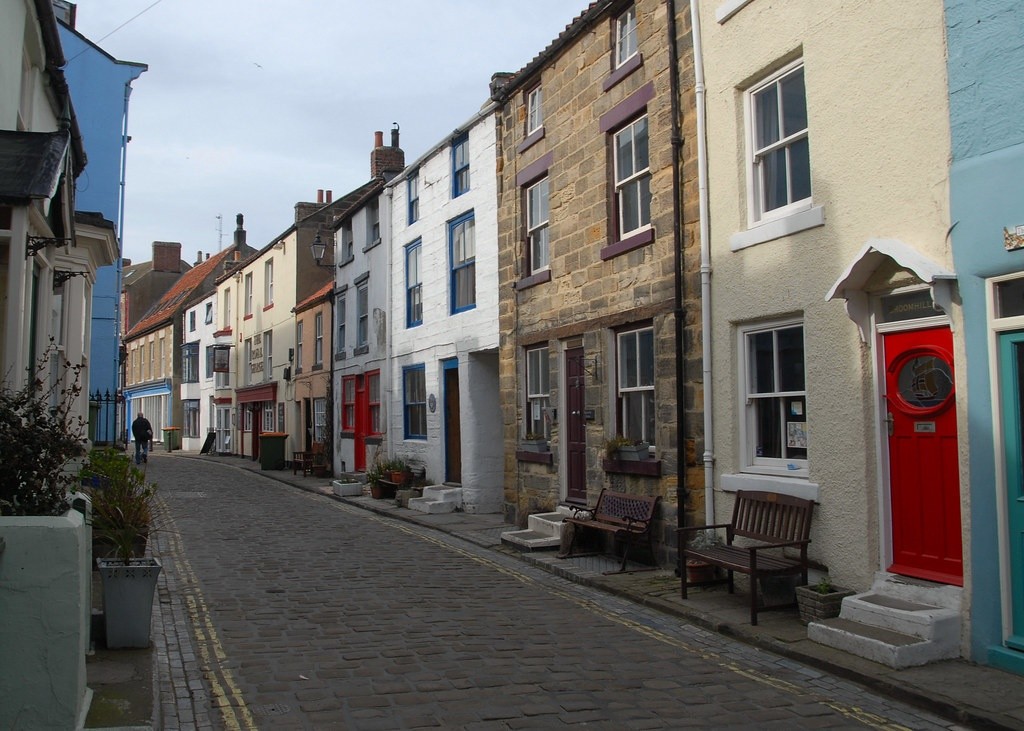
[310, 228, 337, 269]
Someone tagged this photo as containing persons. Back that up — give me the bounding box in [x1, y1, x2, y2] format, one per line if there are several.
[132, 413, 153, 464]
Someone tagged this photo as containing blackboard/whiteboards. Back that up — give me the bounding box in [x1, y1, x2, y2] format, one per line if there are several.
[200, 432, 216, 453]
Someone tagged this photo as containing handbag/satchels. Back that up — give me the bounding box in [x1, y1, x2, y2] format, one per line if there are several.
[144, 432, 150, 440]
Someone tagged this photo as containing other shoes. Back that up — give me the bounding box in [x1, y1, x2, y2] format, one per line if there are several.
[143, 455, 146, 463]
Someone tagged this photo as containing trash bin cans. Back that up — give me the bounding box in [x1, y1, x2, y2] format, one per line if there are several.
[259, 432, 289, 470]
[161, 426, 181, 450]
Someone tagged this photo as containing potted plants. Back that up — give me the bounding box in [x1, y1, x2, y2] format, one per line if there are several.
[364, 458, 433, 508]
[684, 529, 724, 582]
[332, 472, 363, 496]
[606, 435, 650, 463]
[312, 453, 327, 477]
[795, 578, 858, 628]
[1, 334, 187, 731]
[519, 433, 548, 452]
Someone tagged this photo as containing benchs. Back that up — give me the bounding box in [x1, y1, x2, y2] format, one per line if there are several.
[672, 489, 818, 628]
[556, 488, 662, 575]
[291, 442, 325, 477]
